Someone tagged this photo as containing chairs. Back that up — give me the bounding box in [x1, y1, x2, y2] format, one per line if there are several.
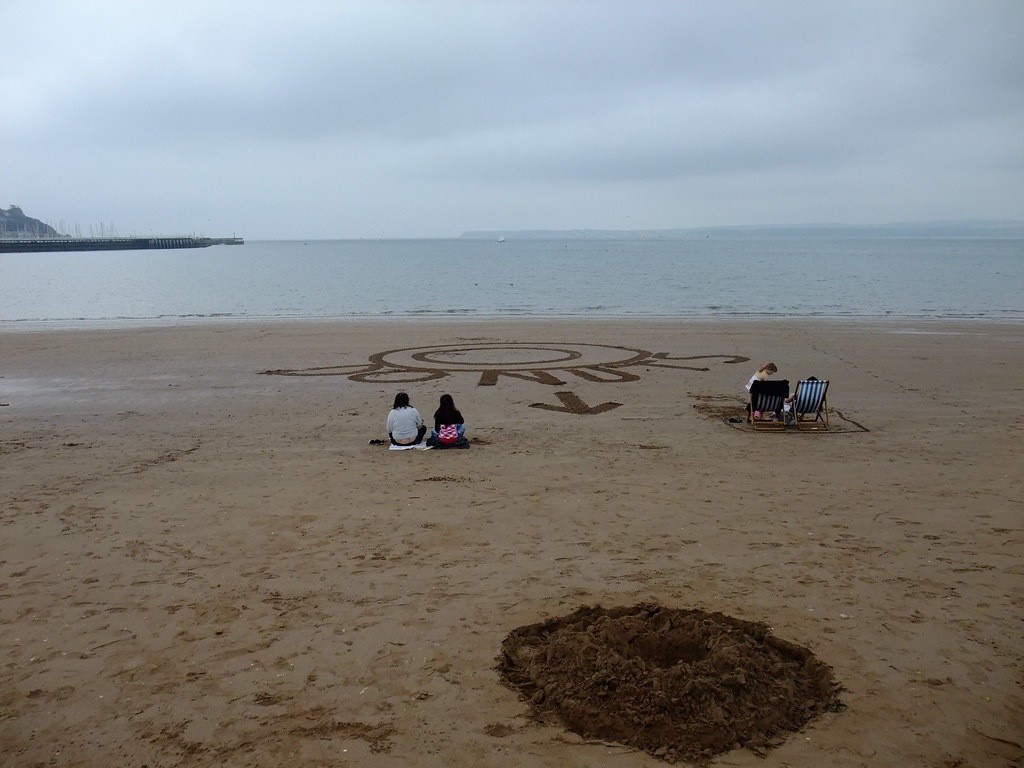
[746, 379, 789, 431]
[792, 380, 830, 431]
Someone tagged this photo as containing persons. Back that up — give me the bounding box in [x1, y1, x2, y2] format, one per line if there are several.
[745, 363, 777, 419]
[386, 393, 428, 446]
[785, 376, 818, 418]
[431, 394, 465, 445]
